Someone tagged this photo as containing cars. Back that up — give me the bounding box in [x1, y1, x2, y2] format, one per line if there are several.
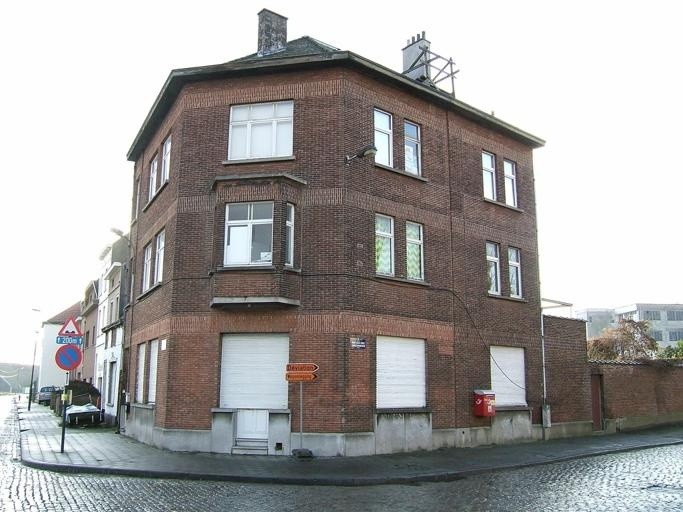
[39, 386, 58, 404]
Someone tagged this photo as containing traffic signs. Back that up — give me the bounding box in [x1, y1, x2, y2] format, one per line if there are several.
[285, 363, 319, 381]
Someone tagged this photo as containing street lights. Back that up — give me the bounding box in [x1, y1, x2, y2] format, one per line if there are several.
[27, 309, 41, 411]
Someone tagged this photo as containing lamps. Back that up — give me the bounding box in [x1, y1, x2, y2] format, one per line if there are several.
[344, 144, 377, 163]
